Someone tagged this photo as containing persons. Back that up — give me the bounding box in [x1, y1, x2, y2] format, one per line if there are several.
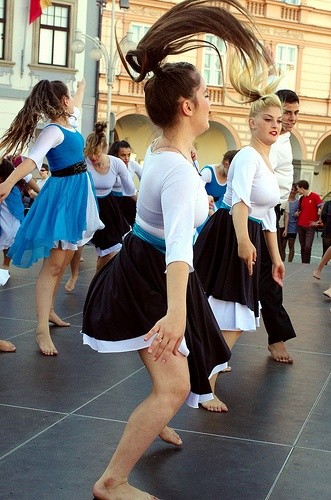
[63, 120, 138, 293]
[193, 150, 239, 244]
[256, 89, 300, 363]
[1, 79, 105, 357]
[191, 93, 287, 414]
[322, 287, 331, 301]
[320, 200, 331, 265]
[79, 61, 218, 500]
[278, 200, 290, 261]
[1, 155, 43, 287]
[10, 156, 41, 214]
[287, 184, 301, 262]
[313, 246, 331, 280]
[104, 138, 145, 243]
[28, 163, 51, 199]
[295, 180, 325, 264]
[0, 340, 16, 353]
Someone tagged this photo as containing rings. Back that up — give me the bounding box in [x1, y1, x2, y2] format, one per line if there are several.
[158, 334, 163, 339]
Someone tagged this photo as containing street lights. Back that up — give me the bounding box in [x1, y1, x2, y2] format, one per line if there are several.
[69, 27, 138, 151]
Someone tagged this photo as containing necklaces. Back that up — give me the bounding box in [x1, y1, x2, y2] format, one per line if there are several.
[154, 138, 201, 176]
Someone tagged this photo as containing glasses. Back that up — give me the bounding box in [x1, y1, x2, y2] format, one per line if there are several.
[40, 170, 46, 172]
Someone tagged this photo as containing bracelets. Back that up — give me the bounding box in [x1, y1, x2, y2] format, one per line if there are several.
[317, 221, 321, 224]
